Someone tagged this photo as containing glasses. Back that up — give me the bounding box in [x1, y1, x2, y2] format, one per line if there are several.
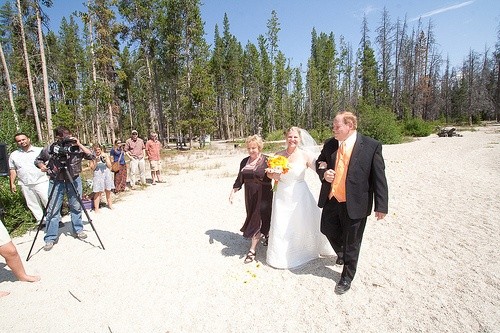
[132, 133, 137, 135]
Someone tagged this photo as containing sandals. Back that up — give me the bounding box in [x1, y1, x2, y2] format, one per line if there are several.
[263, 234, 269, 246]
[245, 250, 256, 262]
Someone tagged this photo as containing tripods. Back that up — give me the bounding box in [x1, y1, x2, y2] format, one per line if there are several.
[26, 162, 106, 262]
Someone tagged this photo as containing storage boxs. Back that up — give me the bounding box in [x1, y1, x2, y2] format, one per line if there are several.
[82, 199, 94, 209]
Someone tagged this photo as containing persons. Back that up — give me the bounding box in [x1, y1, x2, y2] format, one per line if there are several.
[146, 132, 166, 185]
[0, 221, 41, 282]
[266, 126, 327, 269]
[183, 132, 210, 149]
[34, 126, 93, 251]
[229, 135, 273, 262]
[110, 140, 127, 195]
[316, 112, 388, 293]
[124, 130, 150, 189]
[8, 133, 64, 229]
[89, 144, 114, 215]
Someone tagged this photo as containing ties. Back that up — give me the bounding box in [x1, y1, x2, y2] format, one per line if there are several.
[328, 143, 345, 199]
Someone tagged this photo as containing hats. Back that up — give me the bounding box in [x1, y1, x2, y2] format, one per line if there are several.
[132, 130, 137, 133]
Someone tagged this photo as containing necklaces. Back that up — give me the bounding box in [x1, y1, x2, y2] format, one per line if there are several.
[249, 157, 256, 166]
[286, 147, 298, 155]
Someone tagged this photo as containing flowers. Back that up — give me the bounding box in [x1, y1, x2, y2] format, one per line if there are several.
[264, 154, 290, 192]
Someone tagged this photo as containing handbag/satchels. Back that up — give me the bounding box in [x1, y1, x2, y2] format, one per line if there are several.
[111, 162, 119, 172]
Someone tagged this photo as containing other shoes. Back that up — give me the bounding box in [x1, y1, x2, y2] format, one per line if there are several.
[74, 231, 87, 239]
[37, 224, 46, 230]
[59, 221, 64, 228]
[44, 241, 53, 251]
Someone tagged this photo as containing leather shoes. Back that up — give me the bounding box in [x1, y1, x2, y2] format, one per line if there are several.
[335, 279, 350, 294]
[336, 256, 343, 265]
